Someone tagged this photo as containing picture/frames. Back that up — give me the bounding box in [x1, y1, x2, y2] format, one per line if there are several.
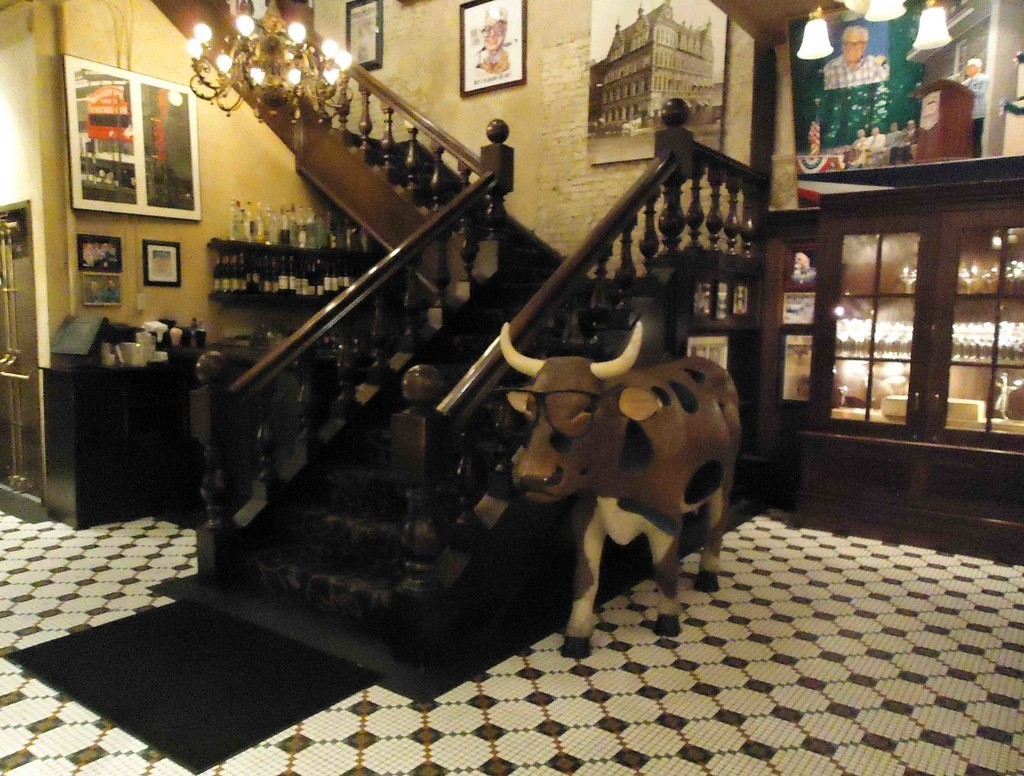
[459, 0, 527, 99]
[76, 233, 123, 273]
[62, 53, 203, 224]
[141, 239, 182, 288]
[345, 0, 384, 72]
[82, 272, 123, 307]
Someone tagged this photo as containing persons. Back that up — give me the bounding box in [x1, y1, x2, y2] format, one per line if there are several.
[823, 25, 889, 89]
[88, 278, 120, 303]
[962, 59, 990, 158]
[82, 242, 116, 267]
[848, 120, 919, 166]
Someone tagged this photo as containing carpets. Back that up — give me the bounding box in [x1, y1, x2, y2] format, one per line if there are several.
[6, 600, 384, 776]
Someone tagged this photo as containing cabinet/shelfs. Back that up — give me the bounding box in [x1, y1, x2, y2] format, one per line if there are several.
[205, 237, 406, 311]
[36, 348, 201, 532]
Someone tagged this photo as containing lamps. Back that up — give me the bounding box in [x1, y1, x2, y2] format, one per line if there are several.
[796, 4, 834, 60]
[912, 0, 953, 50]
[864, 0, 907, 22]
[185, 0, 352, 125]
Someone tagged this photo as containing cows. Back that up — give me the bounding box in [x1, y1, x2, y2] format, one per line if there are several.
[500, 321, 742, 659]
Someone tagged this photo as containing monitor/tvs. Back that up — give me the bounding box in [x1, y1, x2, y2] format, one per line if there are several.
[50, 315, 108, 356]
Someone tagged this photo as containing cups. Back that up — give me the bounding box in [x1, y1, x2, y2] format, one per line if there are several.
[96, 316, 207, 367]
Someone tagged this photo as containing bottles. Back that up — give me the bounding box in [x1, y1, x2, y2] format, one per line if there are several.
[227, 200, 372, 253]
[212, 249, 361, 297]
